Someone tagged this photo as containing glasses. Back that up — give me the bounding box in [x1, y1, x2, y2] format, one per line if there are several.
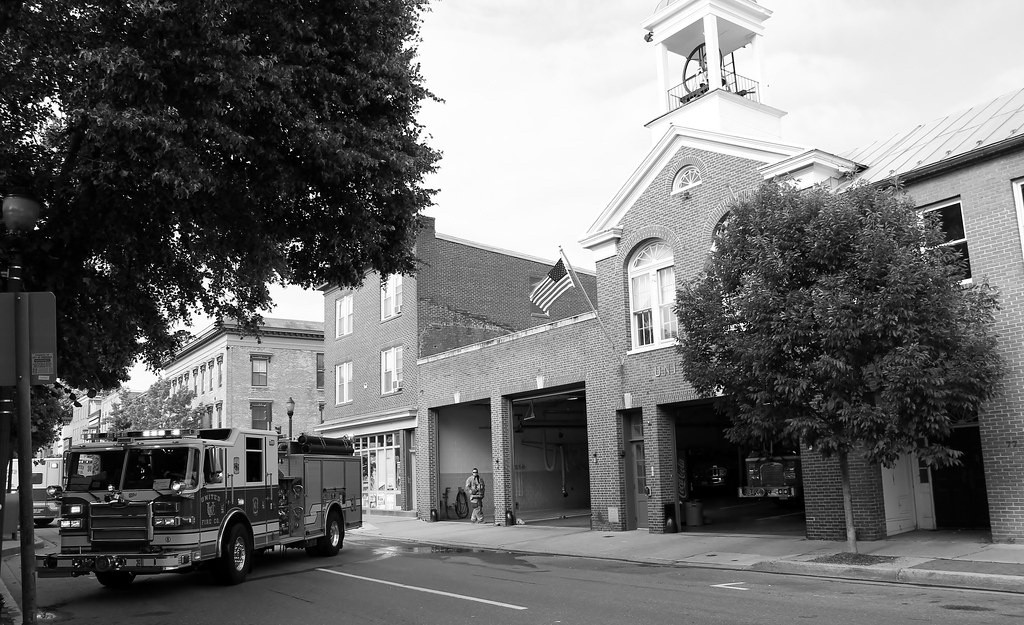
[473, 472, 478, 473]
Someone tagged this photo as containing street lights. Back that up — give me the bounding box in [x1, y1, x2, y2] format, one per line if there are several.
[286, 398, 296, 440]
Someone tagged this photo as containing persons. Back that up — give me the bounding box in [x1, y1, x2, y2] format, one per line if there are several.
[465, 467, 486, 525]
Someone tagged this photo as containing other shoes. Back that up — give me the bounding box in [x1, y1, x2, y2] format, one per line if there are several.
[478, 521, 487, 524]
[471, 519, 475, 525]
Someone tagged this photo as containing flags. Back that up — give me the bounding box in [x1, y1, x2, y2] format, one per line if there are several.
[529, 258, 575, 318]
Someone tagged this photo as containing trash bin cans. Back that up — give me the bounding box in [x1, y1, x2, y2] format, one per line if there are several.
[685, 501, 704, 527]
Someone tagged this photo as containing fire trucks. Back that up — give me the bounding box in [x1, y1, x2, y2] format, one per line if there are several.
[36, 426, 363, 591]
[6, 457, 94, 530]
[738, 440, 803, 501]
